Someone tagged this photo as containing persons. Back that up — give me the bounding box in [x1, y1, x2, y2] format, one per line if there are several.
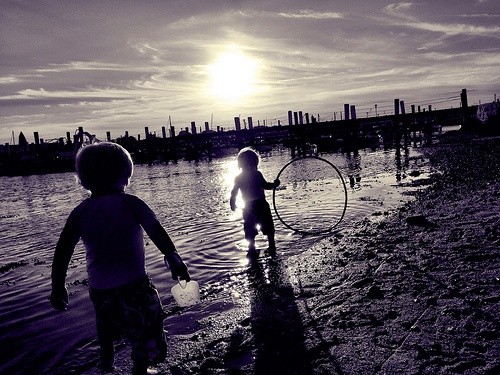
[50, 141, 200, 375]
[229, 147, 281, 255]
